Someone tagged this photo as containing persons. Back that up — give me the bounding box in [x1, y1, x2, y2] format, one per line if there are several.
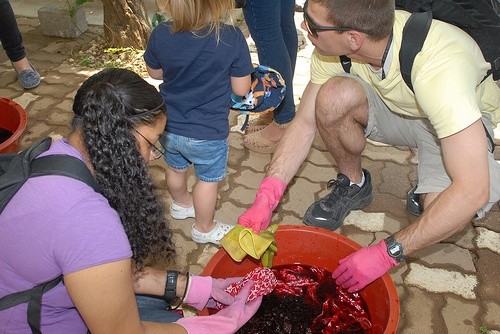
[0, 0, 41, 89]
[237, 0, 500, 293]
[0, 67, 264, 334]
[144, 0, 286, 248]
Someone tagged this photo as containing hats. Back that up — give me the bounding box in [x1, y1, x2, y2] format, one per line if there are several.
[230, 64, 286, 113]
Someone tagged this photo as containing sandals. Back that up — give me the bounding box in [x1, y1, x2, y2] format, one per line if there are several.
[242, 114, 292, 154]
[168, 201, 195, 219]
[190, 220, 234, 245]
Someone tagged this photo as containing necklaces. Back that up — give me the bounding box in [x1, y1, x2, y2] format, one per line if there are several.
[381, 32, 393, 80]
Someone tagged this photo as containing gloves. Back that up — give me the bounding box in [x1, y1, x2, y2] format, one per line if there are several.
[331, 240, 402, 293]
[172, 276, 265, 334]
[238, 176, 288, 234]
[221, 222, 280, 269]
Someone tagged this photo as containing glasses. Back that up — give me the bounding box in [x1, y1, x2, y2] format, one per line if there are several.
[131, 122, 165, 159]
[303, 0, 374, 38]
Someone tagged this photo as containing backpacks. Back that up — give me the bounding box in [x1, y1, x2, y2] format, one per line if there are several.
[0, 137, 96, 311]
[399, 0, 500, 94]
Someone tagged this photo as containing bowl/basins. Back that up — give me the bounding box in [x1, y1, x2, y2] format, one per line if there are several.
[0, 98, 27, 154]
[198, 226, 400, 334]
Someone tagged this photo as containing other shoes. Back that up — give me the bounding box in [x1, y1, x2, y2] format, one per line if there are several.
[11, 57, 41, 89]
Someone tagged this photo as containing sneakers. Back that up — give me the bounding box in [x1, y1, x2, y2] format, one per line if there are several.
[407, 185, 424, 216]
[302, 168, 374, 232]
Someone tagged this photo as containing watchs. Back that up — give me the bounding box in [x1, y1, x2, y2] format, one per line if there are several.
[383, 234, 404, 263]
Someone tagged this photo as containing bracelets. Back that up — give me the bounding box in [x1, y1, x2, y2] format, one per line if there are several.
[163, 270, 189, 310]
